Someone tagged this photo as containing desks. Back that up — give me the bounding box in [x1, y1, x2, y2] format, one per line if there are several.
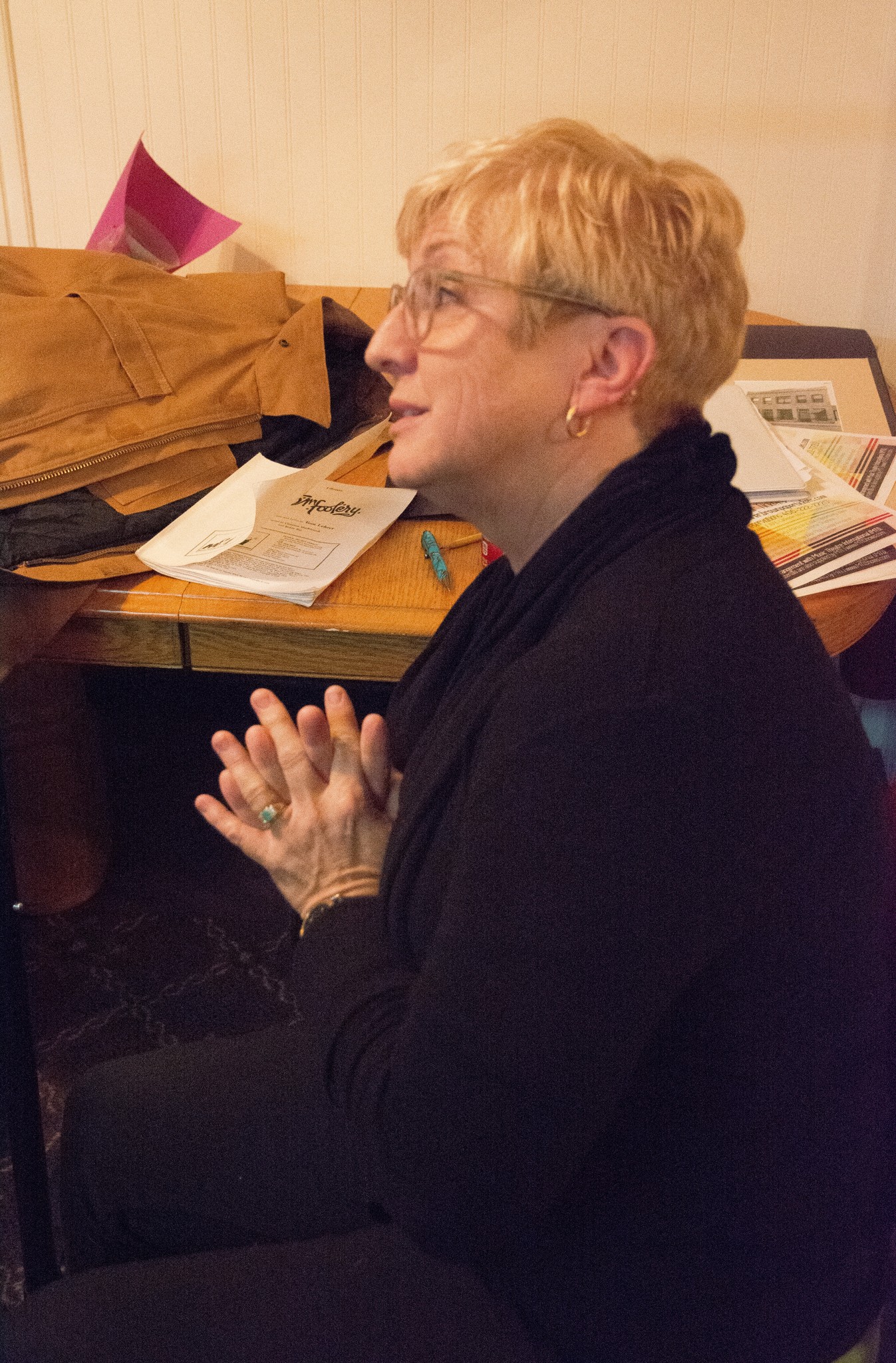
[31, 285, 892, 682]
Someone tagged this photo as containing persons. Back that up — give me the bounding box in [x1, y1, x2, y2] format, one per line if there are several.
[2, 115, 896, 1363]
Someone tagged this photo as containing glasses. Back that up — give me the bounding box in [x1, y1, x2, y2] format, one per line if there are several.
[387, 264, 621, 347]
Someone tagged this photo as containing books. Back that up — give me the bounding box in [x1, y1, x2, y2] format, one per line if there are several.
[697, 380, 811, 500]
[131, 414, 418, 608]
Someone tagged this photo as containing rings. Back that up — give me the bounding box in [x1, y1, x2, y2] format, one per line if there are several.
[256, 801, 285, 824]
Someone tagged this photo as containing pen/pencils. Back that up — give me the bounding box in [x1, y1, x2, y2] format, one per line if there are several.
[421, 531, 451, 593]
[438, 531, 482, 551]
